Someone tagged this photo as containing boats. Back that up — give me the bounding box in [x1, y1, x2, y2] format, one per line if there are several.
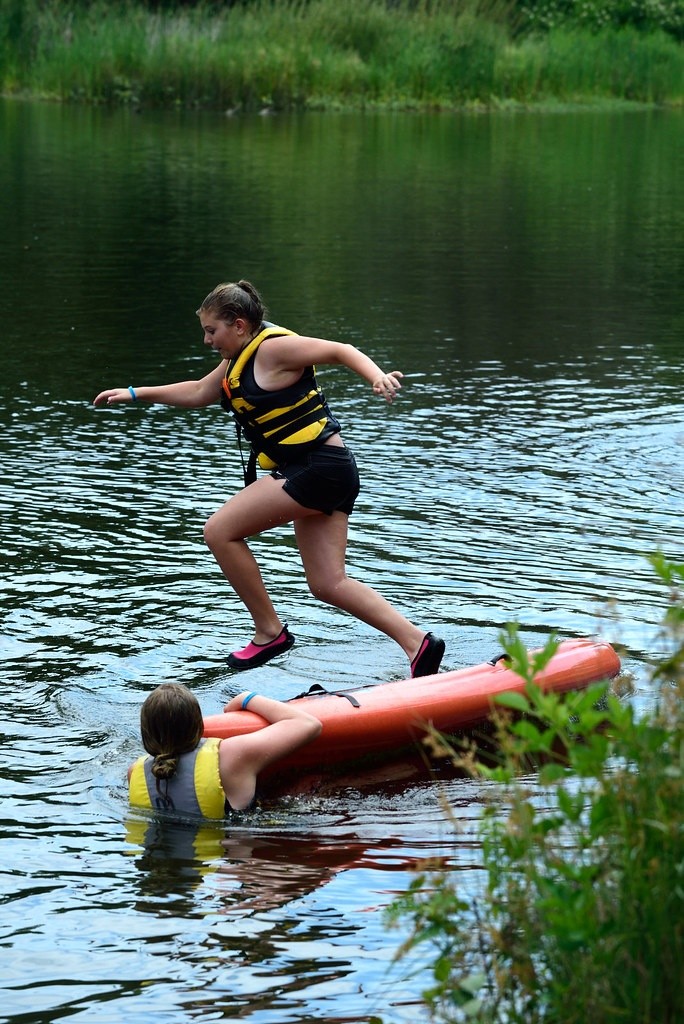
[194, 636, 622, 780]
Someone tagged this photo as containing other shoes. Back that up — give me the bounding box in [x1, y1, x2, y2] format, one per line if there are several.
[410, 632, 445, 678]
[226, 623, 295, 669]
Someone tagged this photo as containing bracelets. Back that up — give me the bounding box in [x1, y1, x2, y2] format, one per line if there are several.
[127, 386, 136, 401]
[242, 691, 257, 710]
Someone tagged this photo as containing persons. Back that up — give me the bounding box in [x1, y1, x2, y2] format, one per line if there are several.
[128, 683, 324, 821]
[93, 279, 445, 678]
